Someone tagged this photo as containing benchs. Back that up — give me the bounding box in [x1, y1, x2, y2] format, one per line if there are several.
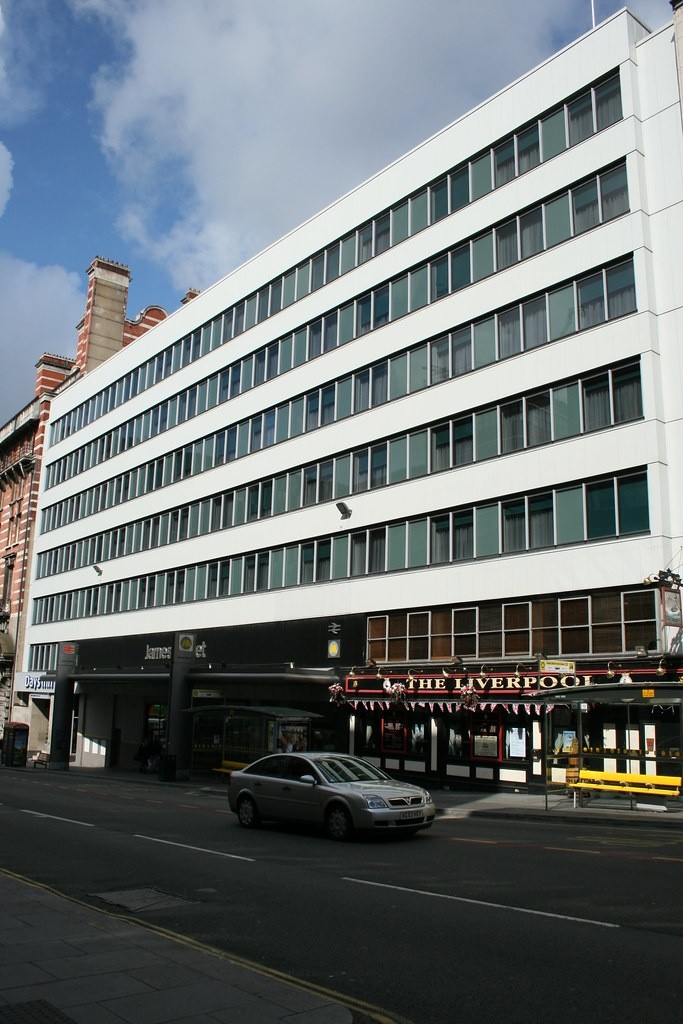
[568, 769, 682, 809]
[212, 759, 250, 783]
[31, 753, 50, 769]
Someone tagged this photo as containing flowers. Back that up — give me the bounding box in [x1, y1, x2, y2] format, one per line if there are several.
[328, 682, 343, 700]
[460, 684, 480, 704]
[387, 683, 407, 701]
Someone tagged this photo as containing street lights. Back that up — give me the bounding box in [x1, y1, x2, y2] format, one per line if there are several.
[569, 701, 590, 808]
[619, 697, 636, 810]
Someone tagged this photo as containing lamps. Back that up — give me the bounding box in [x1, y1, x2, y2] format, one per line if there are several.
[644, 573, 658, 586]
[656, 659, 665, 676]
[533, 652, 548, 661]
[442, 667, 457, 679]
[93, 566, 103, 576]
[451, 656, 463, 664]
[514, 663, 531, 678]
[606, 661, 622, 679]
[376, 666, 392, 680]
[336, 501, 352, 519]
[636, 645, 649, 658]
[364, 658, 377, 667]
[479, 664, 493, 678]
[349, 666, 364, 677]
[407, 669, 423, 682]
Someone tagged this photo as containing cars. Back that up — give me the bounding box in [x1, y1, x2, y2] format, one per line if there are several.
[227, 751, 436, 842]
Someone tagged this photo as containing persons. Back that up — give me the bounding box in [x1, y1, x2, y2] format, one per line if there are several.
[133, 735, 164, 774]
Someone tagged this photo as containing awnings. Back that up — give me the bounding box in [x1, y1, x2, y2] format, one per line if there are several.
[0, 456, 33, 490]
[0, 417, 39, 449]
